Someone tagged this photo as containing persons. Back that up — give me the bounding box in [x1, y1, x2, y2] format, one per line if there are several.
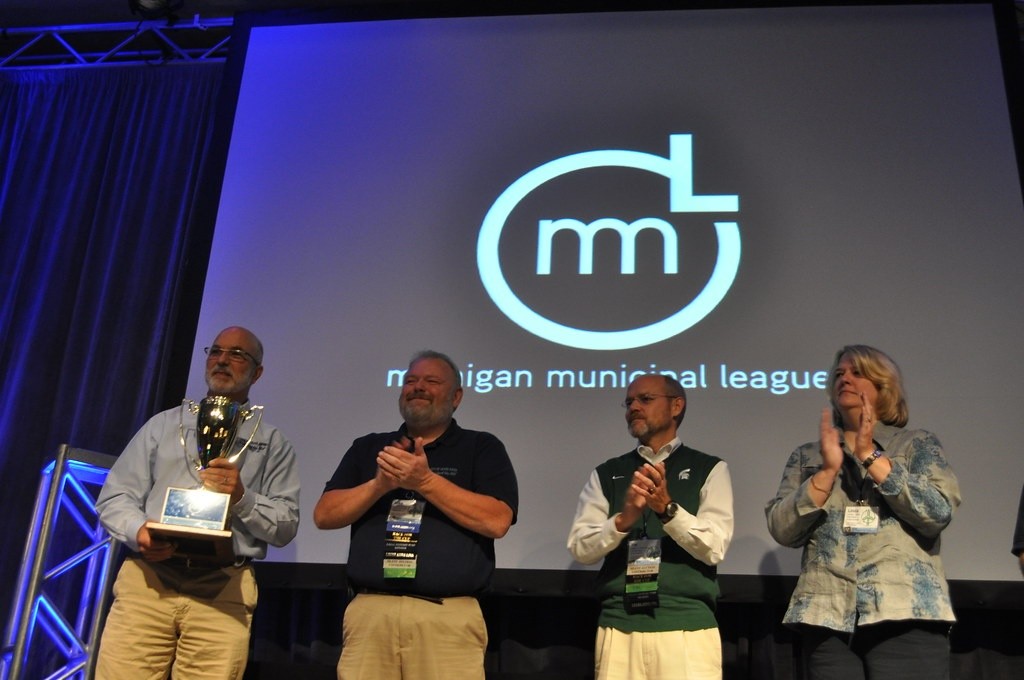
[96, 326, 299, 680]
[566, 374, 733, 679]
[765, 343, 963, 680]
[315, 351, 519, 679]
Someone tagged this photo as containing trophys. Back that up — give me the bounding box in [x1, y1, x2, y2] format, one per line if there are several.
[145, 396, 264, 564]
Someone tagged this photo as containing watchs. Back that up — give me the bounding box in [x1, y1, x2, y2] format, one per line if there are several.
[861, 448, 881, 470]
[654, 501, 679, 522]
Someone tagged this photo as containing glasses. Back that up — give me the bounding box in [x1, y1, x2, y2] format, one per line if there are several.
[204, 347, 258, 365]
[621, 393, 677, 407]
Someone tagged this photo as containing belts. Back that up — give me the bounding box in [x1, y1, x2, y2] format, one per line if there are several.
[351, 586, 445, 604]
[156, 554, 230, 570]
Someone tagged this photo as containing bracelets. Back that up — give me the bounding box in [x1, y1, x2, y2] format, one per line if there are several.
[810, 473, 830, 498]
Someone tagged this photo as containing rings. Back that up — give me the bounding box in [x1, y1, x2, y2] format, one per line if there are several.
[866, 416, 873, 423]
[648, 485, 657, 493]
[223, 476, 231, 483]
